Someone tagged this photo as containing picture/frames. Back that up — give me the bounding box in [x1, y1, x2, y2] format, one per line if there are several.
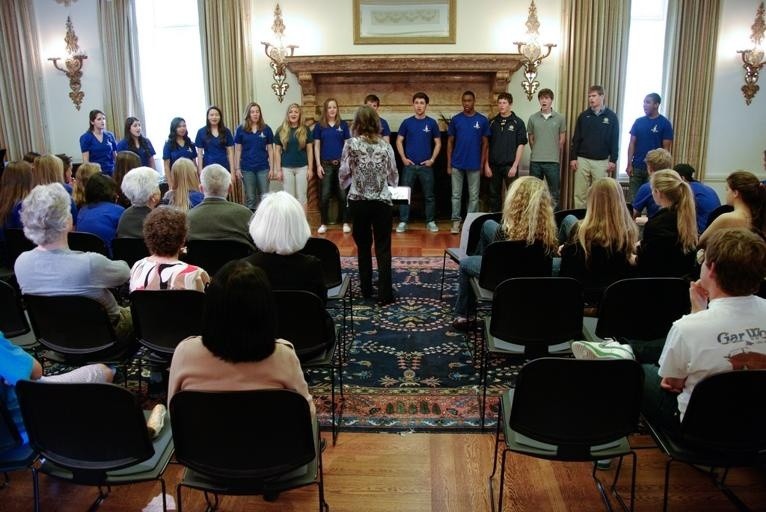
[353, 0, 456, 44]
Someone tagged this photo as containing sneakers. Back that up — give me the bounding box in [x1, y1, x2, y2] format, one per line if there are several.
[317, 224, 328, 234]
[364, 283, 380, 300]
[426, 221, 440, 233]
[570, 337, 637, 363]
[395, 221, 408, 234]
[450, 219, 461, 235]
[592, 457, 616, 472]
[375, 284, 400, 304]
[343, 222, 351, 233]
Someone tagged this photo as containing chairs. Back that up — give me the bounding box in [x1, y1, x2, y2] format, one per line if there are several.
[440, 203, 765, 511]
[0, 182, 351, 512]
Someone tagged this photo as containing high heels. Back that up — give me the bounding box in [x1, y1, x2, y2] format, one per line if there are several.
[452, 316, 469, 332]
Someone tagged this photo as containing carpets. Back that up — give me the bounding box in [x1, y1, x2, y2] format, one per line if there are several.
[5, 247, 676, 433]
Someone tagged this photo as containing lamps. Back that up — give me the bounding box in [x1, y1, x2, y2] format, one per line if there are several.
[46, 17, 88, 112]
[512, 0, 559, 101]
[260, 3, 300, 105]
[731, 3, 766, 104]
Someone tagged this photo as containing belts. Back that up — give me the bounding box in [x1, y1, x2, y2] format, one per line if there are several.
[323, 160, 342, 166]
